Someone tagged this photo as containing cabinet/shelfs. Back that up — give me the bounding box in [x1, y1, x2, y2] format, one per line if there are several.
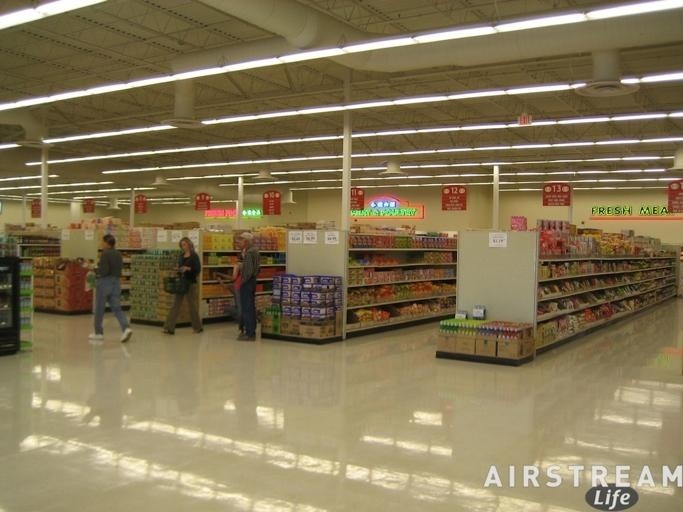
[0, 220, 683, 366]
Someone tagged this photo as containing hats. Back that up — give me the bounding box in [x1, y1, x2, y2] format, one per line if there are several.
[241, 232, 254, 241]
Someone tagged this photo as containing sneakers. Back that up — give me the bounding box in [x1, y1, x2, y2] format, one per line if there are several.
[121, 327, 132, 343]
[194, 327, 203, 333]
[88, 333, 104, 339]
[163, 329, 174, 335]
[237, 333, 256, 341]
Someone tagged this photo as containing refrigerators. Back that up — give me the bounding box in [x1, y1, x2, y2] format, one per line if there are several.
[0, 257, 20, 353]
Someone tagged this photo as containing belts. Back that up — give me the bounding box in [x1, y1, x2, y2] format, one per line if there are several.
[164, 273, 188, 294]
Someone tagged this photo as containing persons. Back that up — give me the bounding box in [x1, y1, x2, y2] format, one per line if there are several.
[213, 231, 258, 340]
[163, 237, 206, 334]
[88, 233, 132, 344]
